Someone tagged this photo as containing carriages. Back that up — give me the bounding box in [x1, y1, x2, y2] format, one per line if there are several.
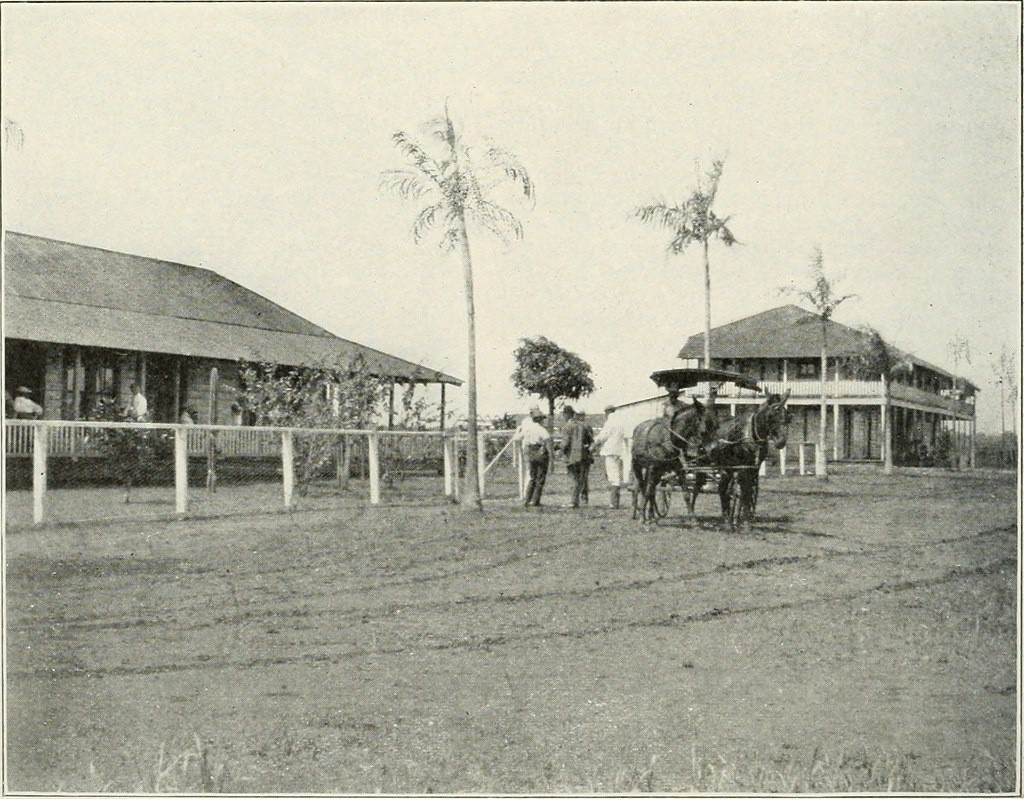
[631, 367, 794, 533]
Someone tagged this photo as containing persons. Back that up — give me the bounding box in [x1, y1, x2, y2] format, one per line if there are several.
[659, 383, 686, 417]
[554, 406, 595, 508]
[13, 386, 43, 420]
[118, 384, 147, 419]
[521, 409, 553, 506]
[595, 406, 628, 510]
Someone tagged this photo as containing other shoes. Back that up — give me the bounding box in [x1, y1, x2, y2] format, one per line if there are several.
[583, 499, 587, 503]
[529, 501, 544, 506]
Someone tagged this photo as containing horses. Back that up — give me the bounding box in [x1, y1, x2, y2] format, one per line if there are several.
[629, 387, 791, 534]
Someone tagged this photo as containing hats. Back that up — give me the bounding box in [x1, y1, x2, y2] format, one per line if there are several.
[561, 405, 576, 413]
[576, 410, 586, 416]
[603, 405, 618, 411]
[666, 382, 685, 393]
[532, 410, 546, 421]
